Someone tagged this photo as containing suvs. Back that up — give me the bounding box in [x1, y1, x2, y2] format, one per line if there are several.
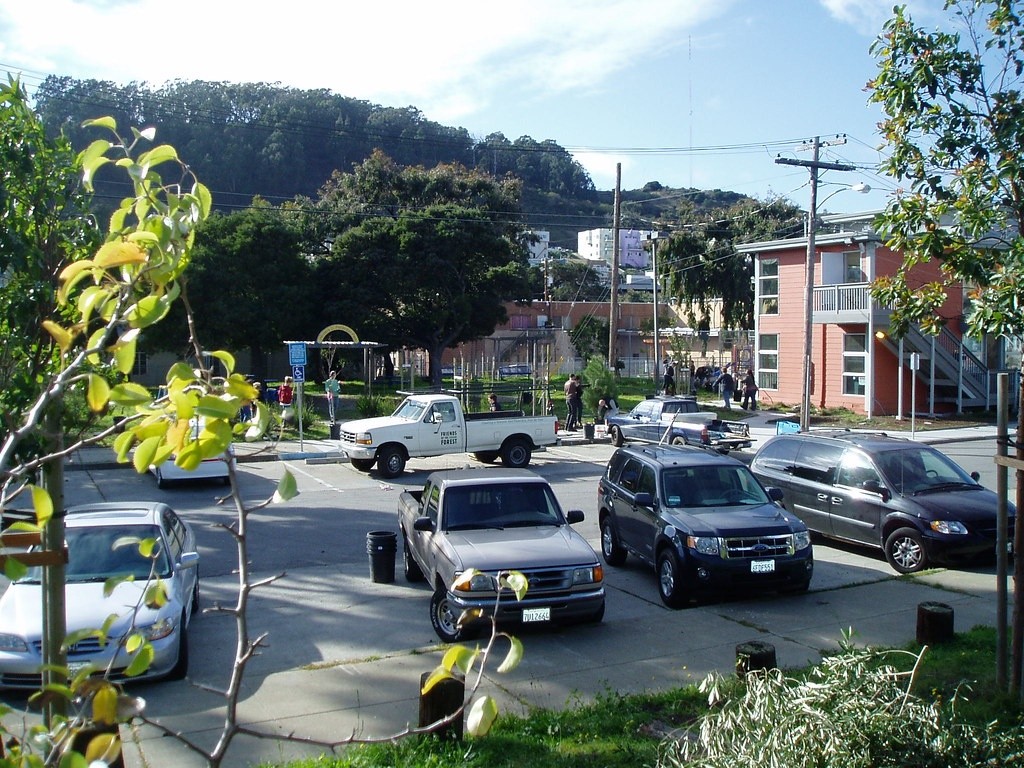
[749, 427, 1016, 574]
[598, 443, 814, 610]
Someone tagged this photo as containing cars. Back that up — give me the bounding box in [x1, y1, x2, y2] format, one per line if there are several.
[1, 502, 200, 694]
[155, 418, 237, 490]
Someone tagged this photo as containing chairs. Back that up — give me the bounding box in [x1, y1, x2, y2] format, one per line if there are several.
[502, 491, 531, 516]
[892, 455, 917, 483]
[449, 495, 475, 524]
[695, 469, 728, 504]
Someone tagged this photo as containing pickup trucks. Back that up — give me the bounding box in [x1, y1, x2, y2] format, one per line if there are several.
[397, 468, 607, 643]
[339, 393, 560, 478]
[603, 398, 757, 456]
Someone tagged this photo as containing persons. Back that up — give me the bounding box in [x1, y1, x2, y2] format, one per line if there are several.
[487, 393, 502, 411]
[661, 359, 758, 409]
[240, 376, 293, 428]
[564, 373, 619, 432]
[325, 370, 341, 424]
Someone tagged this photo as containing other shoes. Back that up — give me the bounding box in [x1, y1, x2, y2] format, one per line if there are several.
[749, 406, 755, 410]
[740, 404, 747, 410]
[566, 427, 577, 431]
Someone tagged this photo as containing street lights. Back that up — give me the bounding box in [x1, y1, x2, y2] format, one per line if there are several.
[639, 231, 670, 396]
[800, 183, 872, 431]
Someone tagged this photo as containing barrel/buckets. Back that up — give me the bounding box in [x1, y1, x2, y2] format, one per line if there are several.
[582, 422, 595, 439]
[366, 530, 397, 583]
